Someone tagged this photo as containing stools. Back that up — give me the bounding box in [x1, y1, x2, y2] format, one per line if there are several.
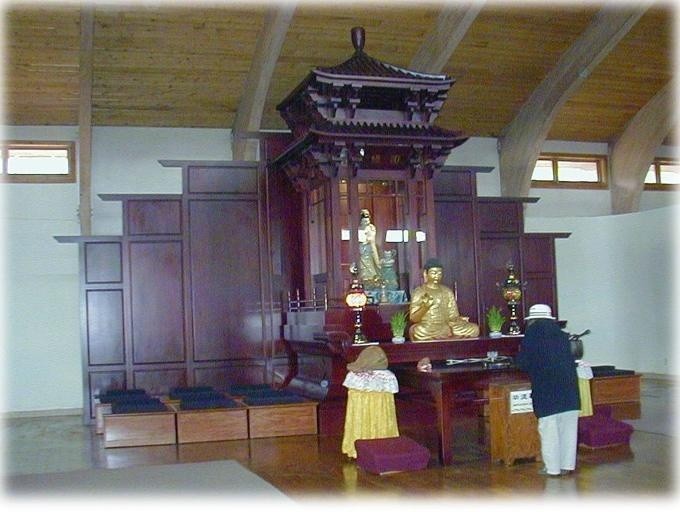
[593, 376, 640, 404]
[94, 389, 320, 448]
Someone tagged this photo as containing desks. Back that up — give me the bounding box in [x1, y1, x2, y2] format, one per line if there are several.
[397, 365, 522, 465]
[313, 329, 531, 465]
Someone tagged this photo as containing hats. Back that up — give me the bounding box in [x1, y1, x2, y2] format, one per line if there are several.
[524, 304, 556, 319]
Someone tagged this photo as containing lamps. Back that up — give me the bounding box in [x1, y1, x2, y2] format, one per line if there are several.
[500, 265, 530, 334]
[346, 281, 369, 344]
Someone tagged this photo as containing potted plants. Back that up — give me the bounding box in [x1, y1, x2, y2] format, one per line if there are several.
[485, 304, 505, 338]
[389, 310, 407, 344]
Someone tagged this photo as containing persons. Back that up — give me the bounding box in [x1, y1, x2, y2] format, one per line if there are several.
[515, 303, 581, 478]
[379, 249, 398, 289]
[407, 258, 480, 340]
[357, 208, 380, 280]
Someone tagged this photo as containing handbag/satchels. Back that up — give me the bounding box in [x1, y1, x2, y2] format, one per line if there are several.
[347, 346, 388, 372]
